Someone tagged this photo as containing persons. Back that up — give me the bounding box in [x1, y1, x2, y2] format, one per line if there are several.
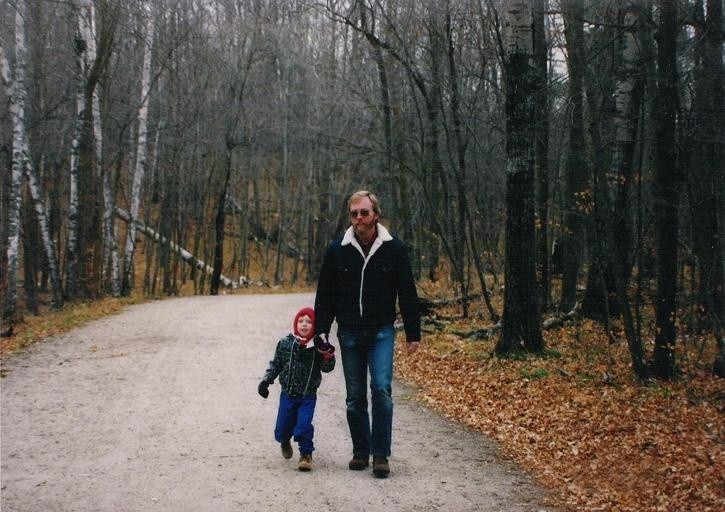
[258, 307, 335, 472]
[314, 189, 421, 478]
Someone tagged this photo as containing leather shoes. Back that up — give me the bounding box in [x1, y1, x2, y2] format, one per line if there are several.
[373, 455, 390, 477]
[281, 441, 293, 458]
[298, 454, 313, 471]
[349, 455, 369, 470]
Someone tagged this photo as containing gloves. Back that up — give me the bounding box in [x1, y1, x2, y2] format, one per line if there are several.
[313, 335, 335, 355]
[258, 380, 270, 399]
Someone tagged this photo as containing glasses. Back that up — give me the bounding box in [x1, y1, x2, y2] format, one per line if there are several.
[349, 209, 370, 218]
